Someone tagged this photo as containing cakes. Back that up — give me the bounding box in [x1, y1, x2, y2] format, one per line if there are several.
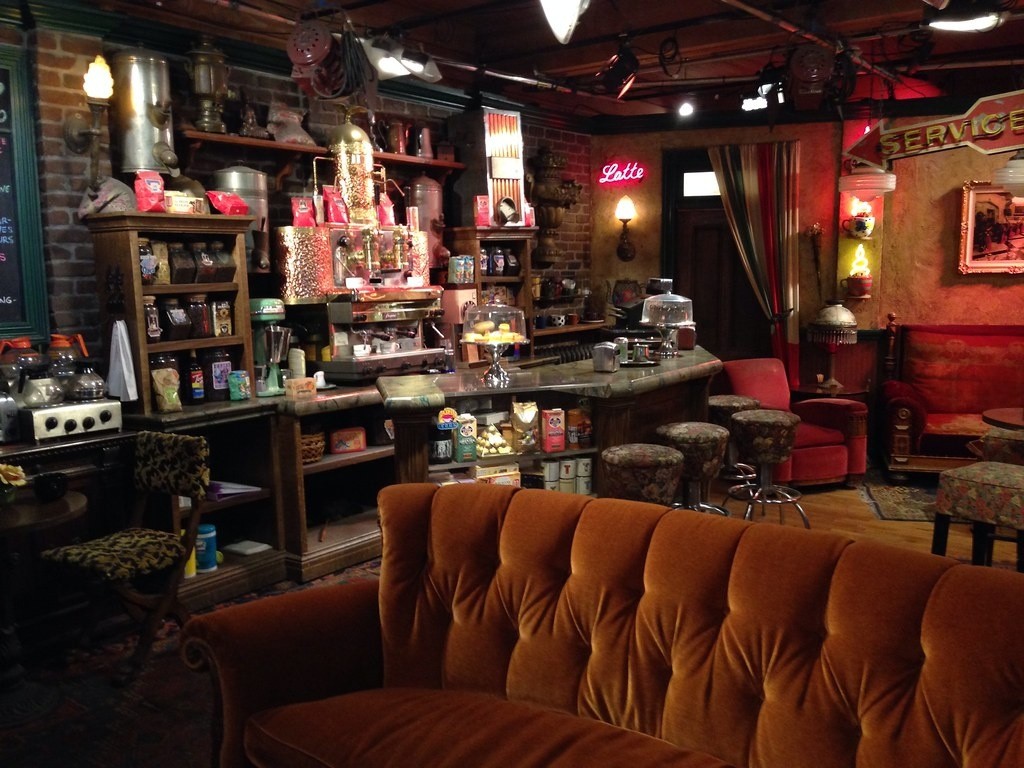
[647, 302, 688, 324]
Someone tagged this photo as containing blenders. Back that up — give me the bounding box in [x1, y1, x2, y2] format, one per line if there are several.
[250, 298, 292, 396]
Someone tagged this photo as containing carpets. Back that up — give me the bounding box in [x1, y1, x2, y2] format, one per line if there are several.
[861, 475, 974, 523]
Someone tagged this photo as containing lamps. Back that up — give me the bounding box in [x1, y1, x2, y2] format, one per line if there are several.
[923, 0, 1009, 32]
[184, 35, 229, 133]
[994, 151, 1024, 184]
[539, 0, 589, 44]
[329, 31, 444, 84]
[63, 57, 113, 197]
[804, 297, 859, 388]
[597, 55, 637, 101]
[613, 193, 639, 261]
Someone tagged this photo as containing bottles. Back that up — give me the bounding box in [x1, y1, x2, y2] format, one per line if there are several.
[143, 296, 161, 343]
[161, 298, 192, 340]
[137, 237, 237, 285]
[206, 348, 232, 400]
[479, 248, 487, 276]
[614, 337, 628, 363]
[489, 246, 504, 275]
[182, 349, 206, 405]
[188, 294, 211, 338]
[211, 301, 232, 336]
[504, 249, 519, 276]
[180, 525, 218, 579]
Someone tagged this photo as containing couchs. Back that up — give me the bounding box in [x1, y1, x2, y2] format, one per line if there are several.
[721, 358, 868, 500]
[881, 311, 1024, 477]
[179, 483, 1024, 768]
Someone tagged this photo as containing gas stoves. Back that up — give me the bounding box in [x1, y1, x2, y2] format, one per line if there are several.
[11, 393, 122, 441]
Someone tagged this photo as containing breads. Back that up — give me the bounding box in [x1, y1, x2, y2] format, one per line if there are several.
[463, 320, 525, 343]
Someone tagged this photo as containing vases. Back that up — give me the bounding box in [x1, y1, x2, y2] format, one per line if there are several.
[0, 485, 17, 506]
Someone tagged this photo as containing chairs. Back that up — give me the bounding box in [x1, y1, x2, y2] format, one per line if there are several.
[42, 432, 210, 689]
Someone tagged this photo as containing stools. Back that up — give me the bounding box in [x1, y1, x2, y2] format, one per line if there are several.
[931, 461, 1024, 572]
[596, 393, 810, 528]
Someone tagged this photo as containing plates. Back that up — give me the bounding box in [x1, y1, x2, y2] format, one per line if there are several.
[316, 384, 337, 390]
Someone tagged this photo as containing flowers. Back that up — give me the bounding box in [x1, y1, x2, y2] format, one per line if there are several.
[0, 462, 28, 491]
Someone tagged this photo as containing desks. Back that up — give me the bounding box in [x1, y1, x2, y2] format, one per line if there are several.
[790, 385, 871, 398]
[980, 407, 1024, 431]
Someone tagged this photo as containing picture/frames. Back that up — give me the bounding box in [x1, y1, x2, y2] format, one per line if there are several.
[957, 179, 1024, 274]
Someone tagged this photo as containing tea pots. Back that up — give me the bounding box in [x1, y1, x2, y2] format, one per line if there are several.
[414, 128, 434, 160]
[604, 277, 647, 308]
[378, 118, 412, 155]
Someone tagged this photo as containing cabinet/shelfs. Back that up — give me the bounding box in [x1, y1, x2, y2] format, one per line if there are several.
[0, 131, 721, 642]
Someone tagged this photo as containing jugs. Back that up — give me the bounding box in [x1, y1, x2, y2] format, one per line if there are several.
[48, 333, 88, 377]
[66, 360, 106, 399]
[0, 336, 43, 378]
[17, 365, 64, 407]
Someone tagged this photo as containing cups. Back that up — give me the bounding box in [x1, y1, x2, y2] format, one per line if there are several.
[535, 317, 547, 329]
[632, 345, 649, 362]
[427, 425, 453, 465]
[551, 315, 565, 327]
[531, 277, 563, 297]
[540, 457, 592, 494]
[568, 314, 580, 324]
[593, 341, 621, 372]
[436, 144, 455, 161]
[843, 216, 876, 237]
[354, 345, 371, 356]
[380, 342, 399, 354]
[840, 277, 873, 295]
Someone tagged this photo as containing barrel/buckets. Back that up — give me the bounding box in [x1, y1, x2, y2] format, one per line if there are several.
[404, 173, 450, 268]
[212, 159, 271, 273]
[108, 48, 173, 175]
